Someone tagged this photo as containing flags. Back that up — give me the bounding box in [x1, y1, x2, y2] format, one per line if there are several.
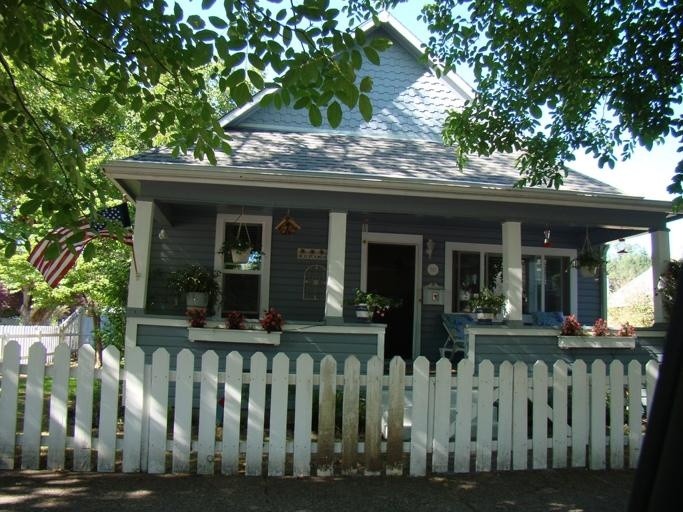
[26, 200, 135, 289]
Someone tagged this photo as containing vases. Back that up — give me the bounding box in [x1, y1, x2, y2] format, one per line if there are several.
[555, 333, 637, 351]
[184, 325, 283, 347]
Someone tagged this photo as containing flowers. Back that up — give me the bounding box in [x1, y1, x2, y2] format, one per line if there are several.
[257, 306, 284, 333]
[559, 314, 582, 335]
[222, 310, 245, 330]
[185, 306, 208, 328]
[615, 320, 634, 336]
[590, 318, 609, 336]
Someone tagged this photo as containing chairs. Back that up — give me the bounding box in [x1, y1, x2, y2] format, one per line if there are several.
[434, 311, 478, 369]
[531, 310, 566, 330]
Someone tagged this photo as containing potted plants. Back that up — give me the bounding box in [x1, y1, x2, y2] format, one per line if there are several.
[160, 261, 221, 315]
[561, 249, 610, 279]
[214, 237, 266, 268]
[463, 287, 505, 325]
[340, 288, 393, 322]
